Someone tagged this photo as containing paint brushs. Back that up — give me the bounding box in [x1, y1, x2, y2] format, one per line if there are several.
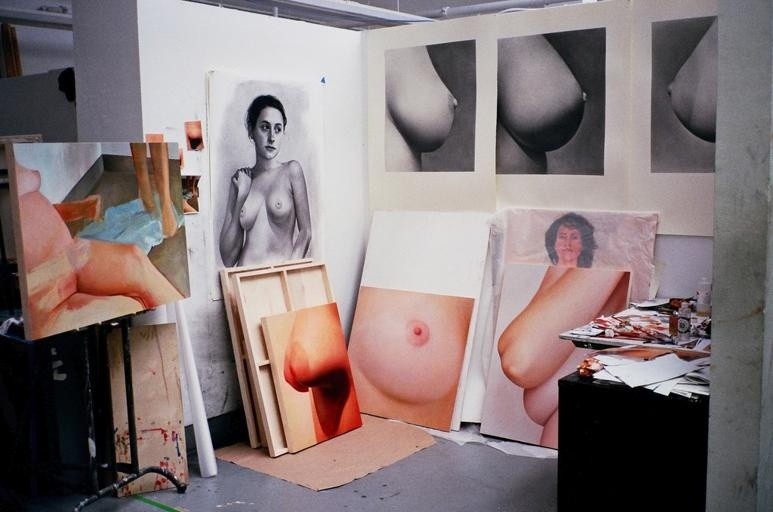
[592, 314, 672, 341]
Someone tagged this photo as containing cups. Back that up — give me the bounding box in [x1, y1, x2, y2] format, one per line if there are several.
[694, 282, 712, 317]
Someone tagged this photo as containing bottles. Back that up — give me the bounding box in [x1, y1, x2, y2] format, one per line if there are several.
[677, 301, 691, 343]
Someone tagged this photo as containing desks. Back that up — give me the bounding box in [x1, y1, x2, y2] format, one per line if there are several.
[553, 368, 708, 512]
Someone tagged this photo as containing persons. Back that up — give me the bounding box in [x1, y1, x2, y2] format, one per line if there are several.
[15, 161, 185, 339]
[497, 36, 587, 174]
[129, 142, 177, 236]
[667, 16, 718, 143]
[386, 46, 458, 172]
[284, 303, 362, 442]
[219, 95, 312, 267]
[497, 213, 630, 449]
[347, 287, 475, 431]
